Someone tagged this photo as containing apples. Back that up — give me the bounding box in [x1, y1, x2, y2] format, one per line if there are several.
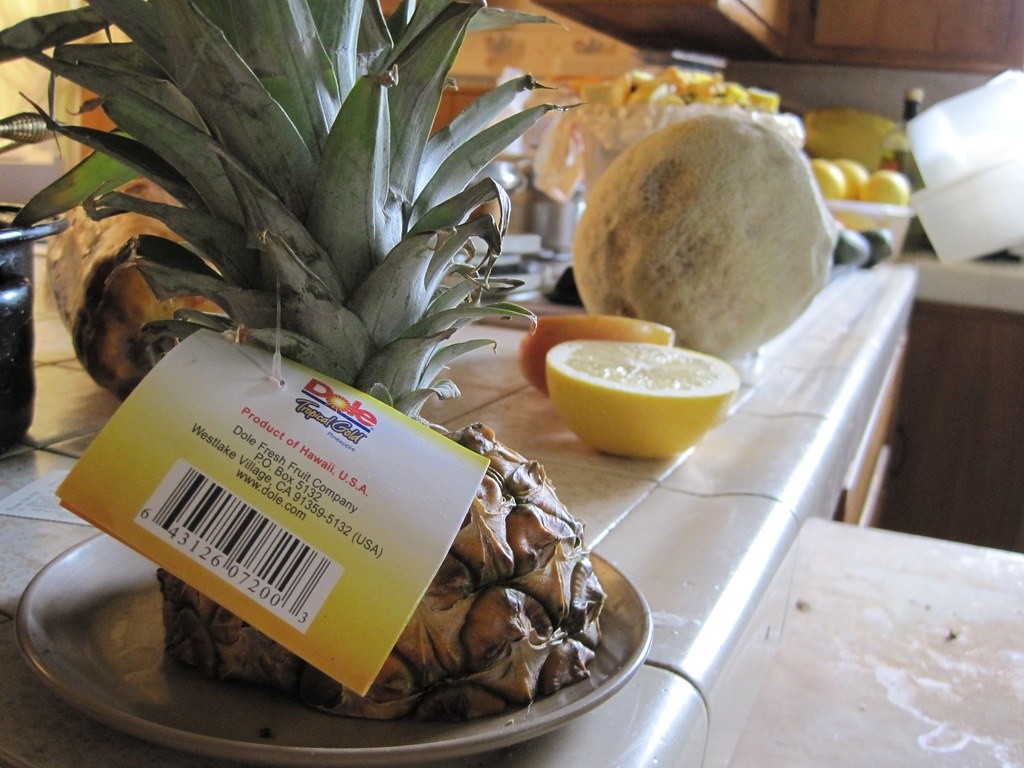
[520, 313, 675, 393]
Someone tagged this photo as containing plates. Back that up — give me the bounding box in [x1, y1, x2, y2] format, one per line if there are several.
[15, 529, 653, 767]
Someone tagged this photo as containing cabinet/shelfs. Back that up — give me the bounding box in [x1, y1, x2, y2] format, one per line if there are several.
[553, 0, 799, 62]
[784, 0, 1024, 76]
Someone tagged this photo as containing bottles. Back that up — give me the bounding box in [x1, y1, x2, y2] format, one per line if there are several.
[878, 86, 933, 252]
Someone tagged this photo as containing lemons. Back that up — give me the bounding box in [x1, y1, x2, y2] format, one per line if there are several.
[811, 158, 910, 229]
[546, 341, 740, 458]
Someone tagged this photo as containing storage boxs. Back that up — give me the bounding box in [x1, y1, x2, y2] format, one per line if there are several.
[905, 151, 1024, 266]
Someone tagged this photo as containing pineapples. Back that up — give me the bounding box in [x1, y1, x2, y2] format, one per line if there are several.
[0, 0, 605, 722]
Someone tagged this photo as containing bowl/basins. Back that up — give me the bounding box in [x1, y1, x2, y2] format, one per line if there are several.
[824, 200, 915, 258]
